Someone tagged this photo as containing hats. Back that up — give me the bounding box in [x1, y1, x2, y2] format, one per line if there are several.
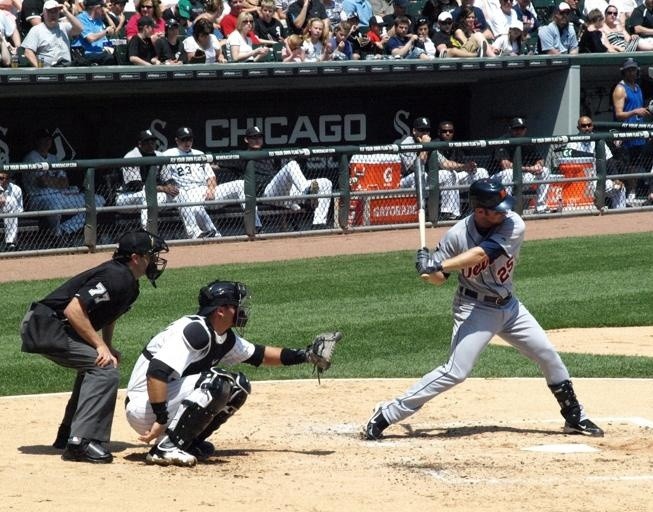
[621, 58, 639, 70]
[439, 12, 454, 22]
[138, 129, 158, 142]
[368, 14, 387, 27]
[556, 2, 571, 13]
[468, 178, 516, 212]
[83, 0, 108, 8]
[245, 126, 264, 137]
[43, 0, 63, 10]
[176, 128, 195, 140]
[412, 117, 432, 131]
[177, 0, 192, 19]
[164, 19, 179, 28]
[509, 20, 524, 32]
[137, 16, 158, 27]
[508, 117, 528, 128]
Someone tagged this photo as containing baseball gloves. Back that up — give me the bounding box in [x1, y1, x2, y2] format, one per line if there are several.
[305, 332, 343, 372]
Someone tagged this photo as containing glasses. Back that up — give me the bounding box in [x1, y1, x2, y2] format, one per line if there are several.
[579, 123, 592, 128]
[415, 128, 429, 132]
[439, 130, 453, 133]
[141, 4, 154, 9]
[605, 12, 617, 17]
[242, 20, 253, 25]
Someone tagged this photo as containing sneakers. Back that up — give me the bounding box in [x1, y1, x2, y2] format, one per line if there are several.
[52, 425, 113, 464]
[306, 181, 320, 209]
[309, 224, 331, 230]
[198, 228, 215, 238]
[533, 204, 550, 214]
[563, 412, 605, 436]
[438, 41, 504, 59]
[5, 233, 73, 252]
[366, 401, 392, 440]
[146, 441, 214, 467]
[255, 225, 265, 234]
[438, 212, 460, 221]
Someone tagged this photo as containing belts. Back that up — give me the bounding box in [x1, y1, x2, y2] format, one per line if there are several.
[29, 301, 76, 333]
[456, 286, 512, 306]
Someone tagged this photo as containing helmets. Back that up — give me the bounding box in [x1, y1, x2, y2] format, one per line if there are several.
[117, 230, 170, 282]
[197, 278, 253, 337]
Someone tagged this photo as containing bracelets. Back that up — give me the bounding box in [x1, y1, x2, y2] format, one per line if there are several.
[151, 401, 169, 424]
[280, 348, 309, 366]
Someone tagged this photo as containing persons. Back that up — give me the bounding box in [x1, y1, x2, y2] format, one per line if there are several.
[20, 231, 169, 464]
[367, 177, 605, 441]
[124, 280, 342, 467]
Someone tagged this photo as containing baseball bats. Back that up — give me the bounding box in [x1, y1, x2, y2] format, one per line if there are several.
[414, 155, 429, 280]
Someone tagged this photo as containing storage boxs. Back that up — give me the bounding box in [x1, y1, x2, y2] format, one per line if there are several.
[528, 173, 564, 211]
[346, 152, 403, 194]
[331, 188, 423, 228]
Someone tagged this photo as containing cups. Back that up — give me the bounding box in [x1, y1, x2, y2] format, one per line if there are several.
[9, 54, 19, 68]
[36, 54, 45, 69]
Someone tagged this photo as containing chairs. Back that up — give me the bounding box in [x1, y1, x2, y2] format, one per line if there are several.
[11, 0, 590, 69]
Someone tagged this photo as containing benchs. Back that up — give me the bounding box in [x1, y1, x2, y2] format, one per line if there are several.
[422, 156, 538, 222]
[99, 164, 311, 244]
[0, 215, 101, 252]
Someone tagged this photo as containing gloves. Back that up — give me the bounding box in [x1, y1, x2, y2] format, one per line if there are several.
[416, 247, 440, 274]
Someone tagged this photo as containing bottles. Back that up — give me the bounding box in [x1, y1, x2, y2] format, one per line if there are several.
[381, 26, 388, 39]
[366, 54, 401, 68]
